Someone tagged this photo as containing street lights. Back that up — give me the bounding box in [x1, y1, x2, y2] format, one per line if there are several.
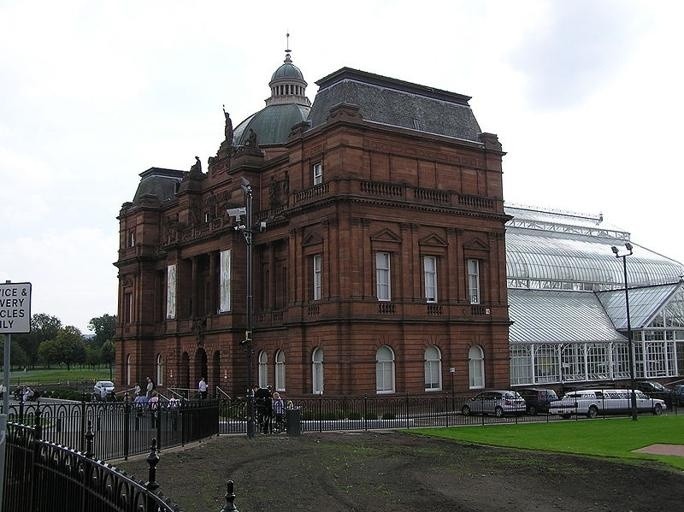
[610, 241, 642, 420]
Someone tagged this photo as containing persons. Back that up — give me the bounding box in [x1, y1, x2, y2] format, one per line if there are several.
[223, 108, 234, 146]
[246, 129, 256, 149]
[99, 376, 181, 410]
[197, 375, 208, 400]
[191, 156, 201, 173]
[282, 170, 289, 196]
[0, 384, 34, 404]
[266, 175, 278, 200]
[251, 385, 295, 434]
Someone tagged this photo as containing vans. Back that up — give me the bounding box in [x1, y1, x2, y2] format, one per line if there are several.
[516, 387, 559, 417]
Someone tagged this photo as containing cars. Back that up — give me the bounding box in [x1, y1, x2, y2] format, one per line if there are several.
[628, 380, 684, 409]
[548, 389, 668, 419]
[460, 389, 529, 418]
[93, 380, 115, 398]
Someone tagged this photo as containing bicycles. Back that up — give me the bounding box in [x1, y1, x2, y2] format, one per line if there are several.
[223, 399, 286, 434]
